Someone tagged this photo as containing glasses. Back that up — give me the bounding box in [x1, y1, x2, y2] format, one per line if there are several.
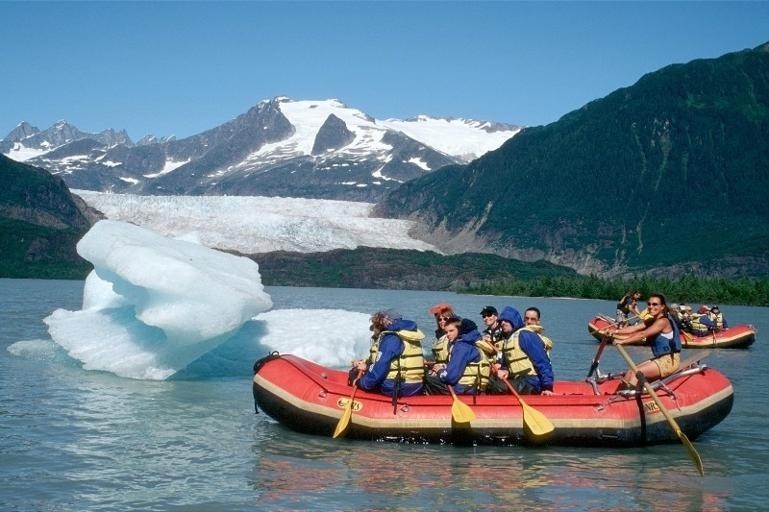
[648, 302, 657, 306]
[438, 317, 449, 322]
[482, 312, 493, 318]
[525, 317, 536, 320]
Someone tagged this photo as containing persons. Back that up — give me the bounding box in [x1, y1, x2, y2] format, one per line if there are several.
[640, 302, 729, 337]
[615, 289, 643, 329]
[348, 303, 555, 397]
[604, 292, 682, 394]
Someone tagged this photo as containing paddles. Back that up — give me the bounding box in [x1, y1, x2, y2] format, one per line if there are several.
[448, 384, 476, 423]
[680, 328, 697, 343]
[588, 315, 640, 335]
[492, 362, 555, 436]
[713, 329, 717, 346]
[612, 338, 704, 476]
[332, 367, 366, 438]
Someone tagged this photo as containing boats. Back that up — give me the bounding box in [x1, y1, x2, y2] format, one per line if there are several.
[252, 351, 734, 448]
[587, 311, 757, 351]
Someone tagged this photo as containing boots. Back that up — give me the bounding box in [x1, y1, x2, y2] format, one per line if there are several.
[604, 379, 637, 395]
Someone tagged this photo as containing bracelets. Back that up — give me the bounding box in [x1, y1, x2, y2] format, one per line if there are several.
[616, 328, 620, 334]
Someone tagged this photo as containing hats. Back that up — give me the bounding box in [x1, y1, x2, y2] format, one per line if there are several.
[480, 306, 497, 314]
[700, 305, 711, 313]
[383, 309, 400, 321]
[671, 304, 678, 308]
[713, 306, 718, 309]
[679, 305, 686, 311]
[656, 293, 670, 312]
[687, 306, 692, 311]
[429, 304, 451, 314]
